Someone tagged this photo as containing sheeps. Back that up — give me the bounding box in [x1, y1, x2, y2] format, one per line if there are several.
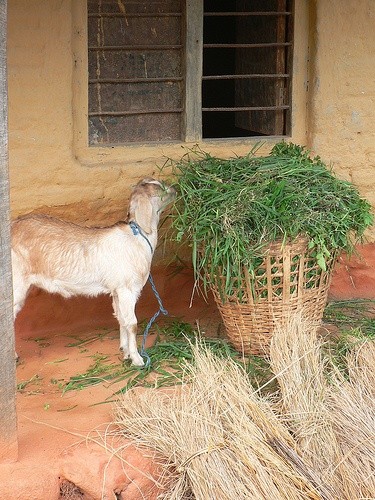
[10, 177, 178, 370]
